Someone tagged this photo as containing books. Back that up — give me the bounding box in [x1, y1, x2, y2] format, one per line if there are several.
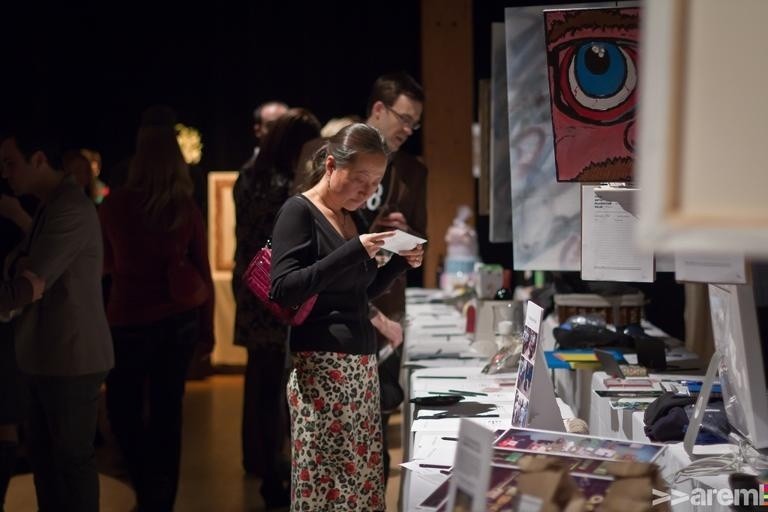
[593, 361, 729, 417]
[418, 422, 668, 512]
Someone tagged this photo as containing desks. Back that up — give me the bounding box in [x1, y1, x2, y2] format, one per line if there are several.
[400, 286, 768, 512]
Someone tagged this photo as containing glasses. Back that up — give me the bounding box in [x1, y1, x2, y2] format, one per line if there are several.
[388, 109, 421, 134]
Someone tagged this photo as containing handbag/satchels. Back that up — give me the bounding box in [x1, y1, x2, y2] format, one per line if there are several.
[243, 249, 319, 327]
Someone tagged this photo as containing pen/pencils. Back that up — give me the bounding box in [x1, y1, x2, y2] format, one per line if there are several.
[442, 437, 458, 441]
[419, 464, 451, 468]
[429, 390, 488, 397]
[501, 383, 515, 386]
[424, 325, 457, 327]
[417, 376, 466, 379]
[431, 333, 464, 336]
